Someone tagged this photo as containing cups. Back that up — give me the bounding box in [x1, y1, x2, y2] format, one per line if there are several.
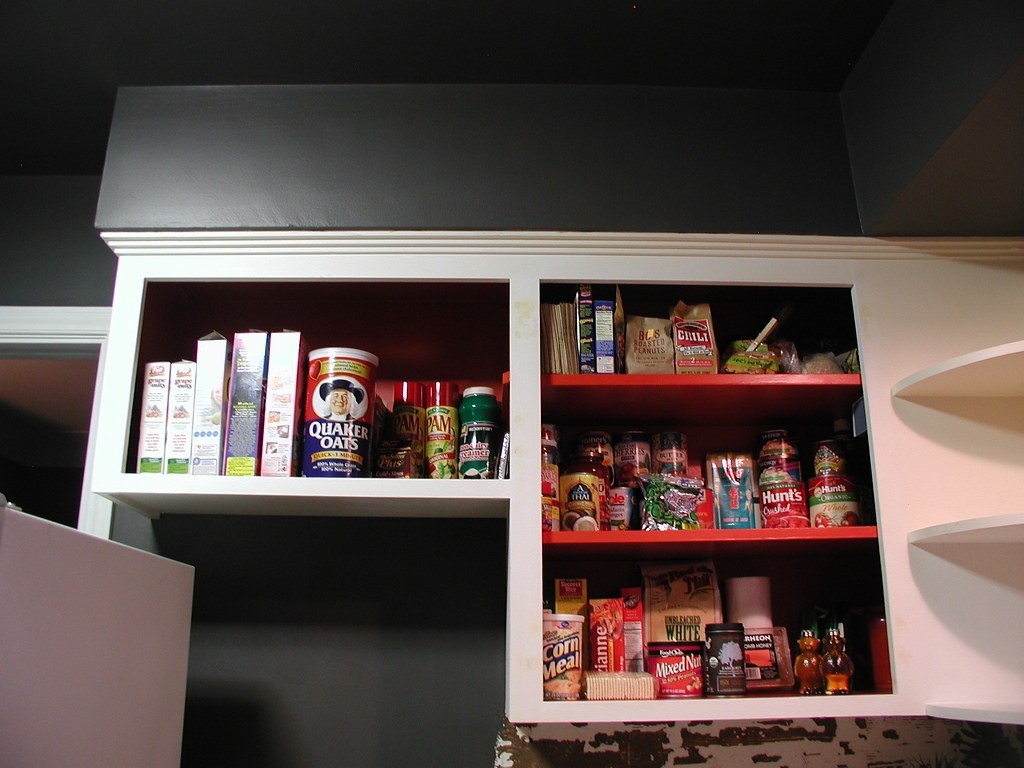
[704, 623, 747, 695]
[728, 575, 772, 630]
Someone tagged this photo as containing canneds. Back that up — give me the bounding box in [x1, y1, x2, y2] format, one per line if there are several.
[298, 347, 378, 477]
[704, 623, 747, 697]
[542, 613, 585, 699]
[458, 420, 499, 479]
[543, 421, 866, 531]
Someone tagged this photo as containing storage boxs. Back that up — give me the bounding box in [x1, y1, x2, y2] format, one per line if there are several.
[137, 361, 171, 477]
[588, 598, 625, 671]
[223, 327, 268, 476]
[261, 329, 311, 477]
[191, 332, 235, 475]
[164, 358, 196, 474]
[577, 283, 595, 375]
[554, 576, 588, 615]
[621, 585, 645, 674]
[594, 300, 614, 373]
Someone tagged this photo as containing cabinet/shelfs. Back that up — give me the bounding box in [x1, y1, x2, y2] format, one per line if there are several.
[49, 229, 1024, 727]
